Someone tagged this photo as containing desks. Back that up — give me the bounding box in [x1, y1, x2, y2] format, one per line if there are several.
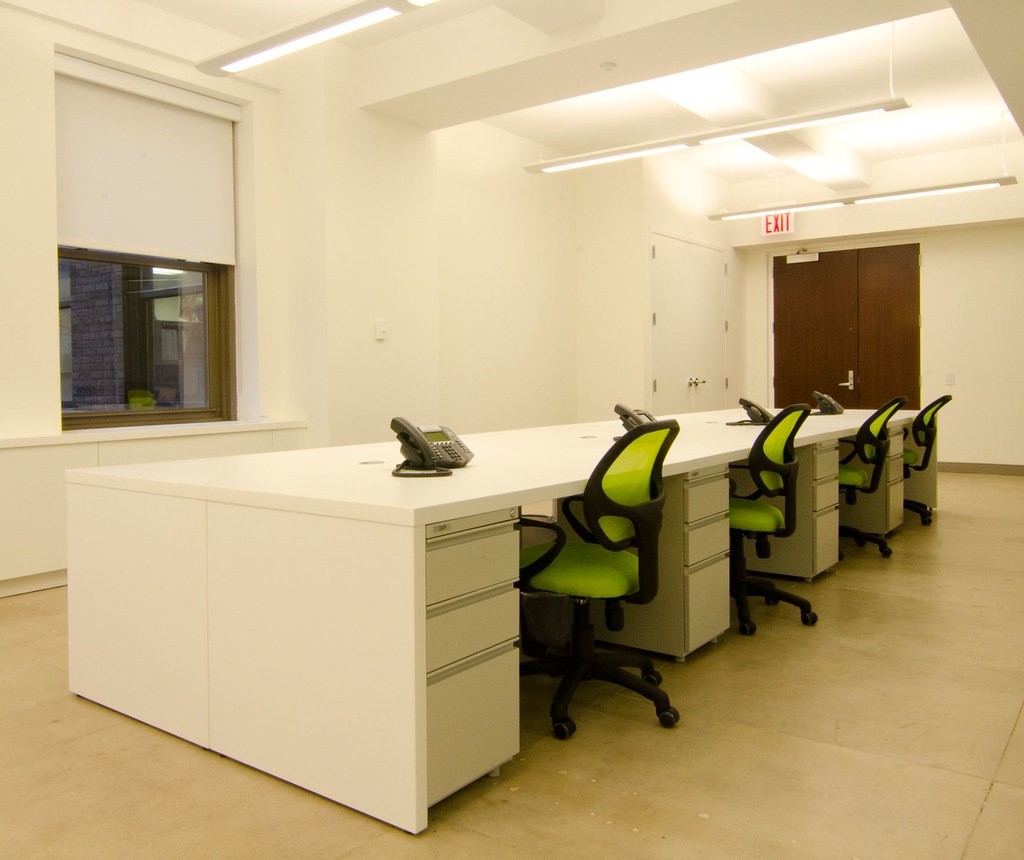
[64, 407, 937, 834]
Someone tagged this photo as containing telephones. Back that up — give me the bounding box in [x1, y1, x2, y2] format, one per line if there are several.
[390, 416, 474, 468]
[614, 403, 658, 431]
[738, 397, 774, 425]
[813, 390, 844, 414]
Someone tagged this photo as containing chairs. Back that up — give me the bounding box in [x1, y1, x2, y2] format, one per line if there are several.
[729, 403, 819, 636]
[518, 420, 681, 739]
[903, 394, 952, 526]
[838, 396, 910, 558]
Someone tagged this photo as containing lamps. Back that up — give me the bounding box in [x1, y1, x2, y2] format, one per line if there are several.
[194, 0, 444, 80]
[706, 147, 1019, 221]
[522, 25, 913, 175]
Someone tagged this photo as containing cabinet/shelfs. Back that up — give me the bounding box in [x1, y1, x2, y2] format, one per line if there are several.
[731, 439, 840, 581]
[839, 428, 903, 539]
[592, 463, 731, 662]
[426, 504, 520, 809]
